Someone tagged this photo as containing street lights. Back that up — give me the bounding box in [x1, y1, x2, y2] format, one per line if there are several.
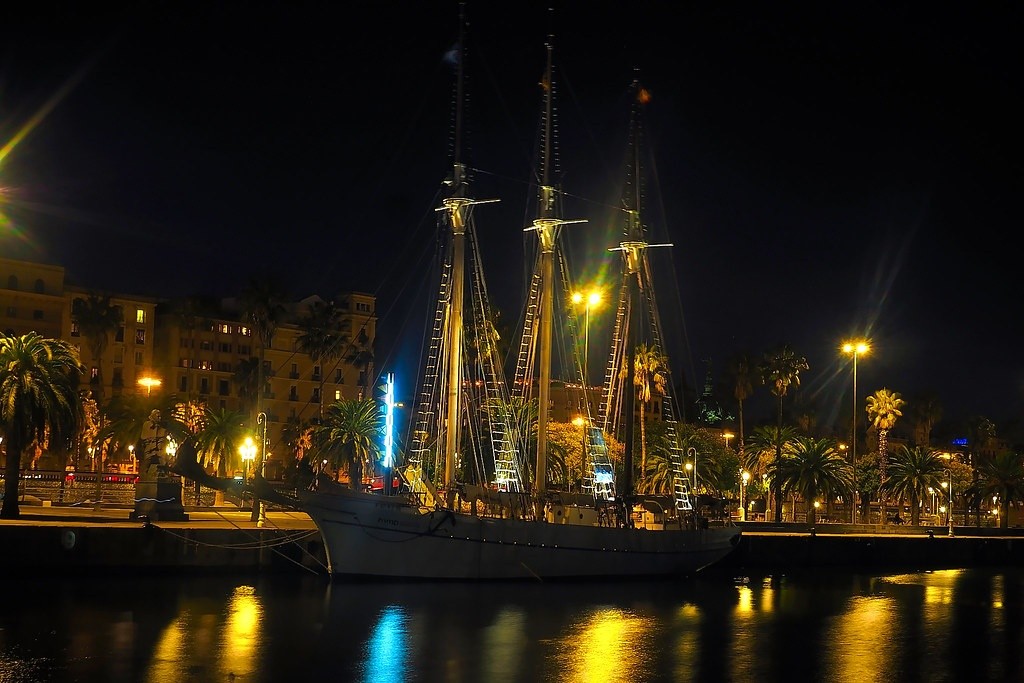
[942, 468, 956, 537]
[838, 339, 872, 522]
[240, 410, 267, 522]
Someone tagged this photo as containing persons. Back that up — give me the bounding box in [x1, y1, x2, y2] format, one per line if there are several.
[141, 408, 167, 463]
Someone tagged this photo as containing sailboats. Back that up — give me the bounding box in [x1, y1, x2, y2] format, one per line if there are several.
[156, 1, 741, 581]
